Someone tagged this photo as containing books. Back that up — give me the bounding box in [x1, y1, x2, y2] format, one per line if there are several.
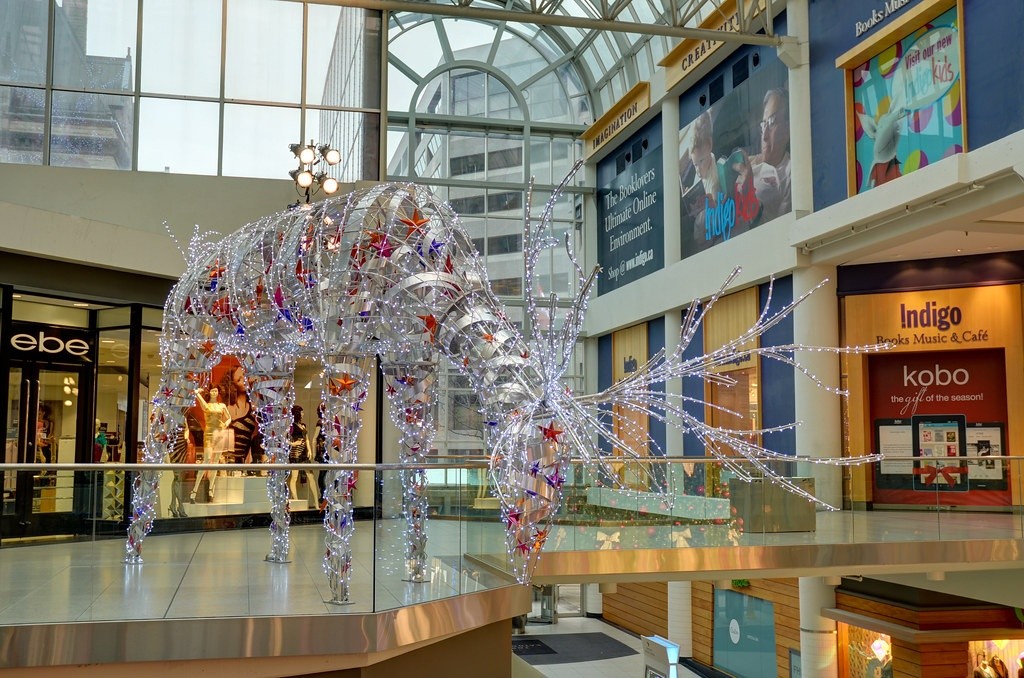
[679, 176, 707, 219]
[717, 150, 746, 205]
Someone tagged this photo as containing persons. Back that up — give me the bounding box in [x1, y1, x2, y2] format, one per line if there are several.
[94, 420, 107, 466]
[167, 404, 190, 517]
[108, 434, 117, 459]
[974, 658, 1024, 678]
[222, 364, 259, 464]
[880, 654, 893, 678]
[682, 85, 792, 259]
[311, 403, 326, 501]
[190, 388, 231, 504]
[33, 406, 54, 486]
[289, 406, 323, 510]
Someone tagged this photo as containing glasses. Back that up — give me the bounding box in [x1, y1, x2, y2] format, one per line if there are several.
[760, 105, 786, 132]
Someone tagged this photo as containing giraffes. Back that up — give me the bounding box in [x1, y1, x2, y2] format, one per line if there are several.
[122, 159, 899, 605]
